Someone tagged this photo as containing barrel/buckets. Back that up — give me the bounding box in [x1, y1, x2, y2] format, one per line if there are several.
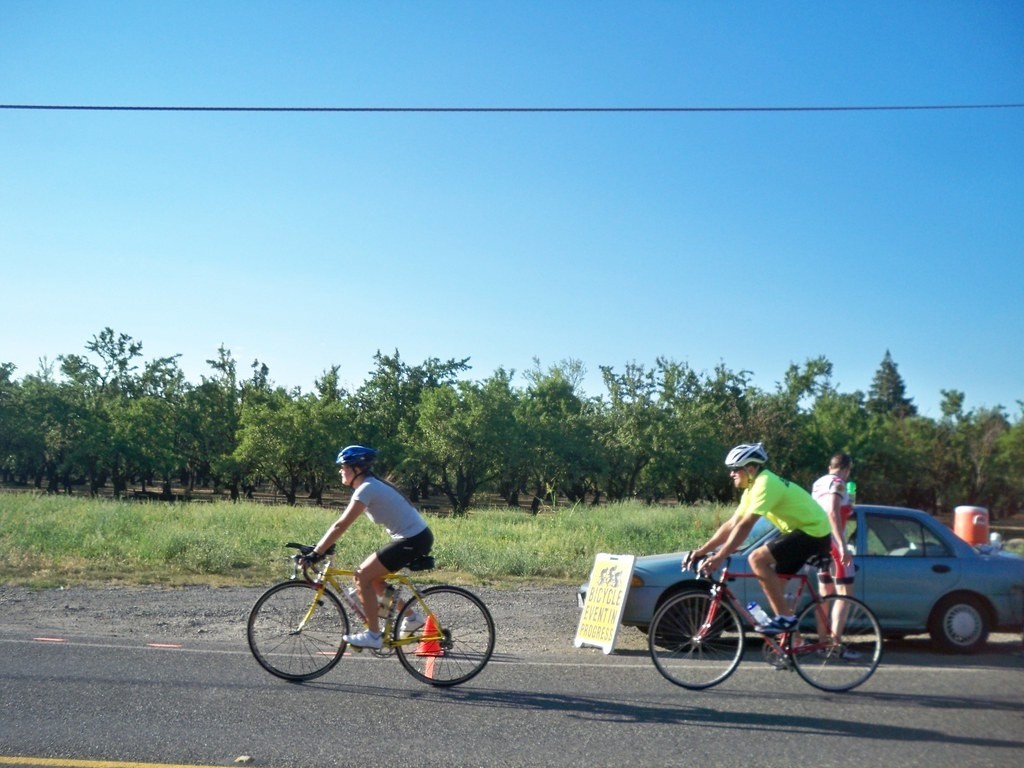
[954, 506, 989, 549]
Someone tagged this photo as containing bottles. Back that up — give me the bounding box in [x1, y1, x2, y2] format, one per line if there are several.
[377, 584, 395, 617]
[847, 480, 856, 506]
[747, 600, 773, 627]
[783, 591, 795, 614]
[348, 586, 366, 615]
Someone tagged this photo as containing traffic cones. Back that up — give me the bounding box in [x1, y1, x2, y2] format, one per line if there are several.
[415, 614, 445, 658]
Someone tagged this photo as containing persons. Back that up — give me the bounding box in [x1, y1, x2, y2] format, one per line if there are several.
[678, 440, 835, 650]
[296, 444, 435, 651]
[805, 451, 867, 663]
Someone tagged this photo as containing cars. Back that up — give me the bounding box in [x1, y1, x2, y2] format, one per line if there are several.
[577, 503, 1024, 653]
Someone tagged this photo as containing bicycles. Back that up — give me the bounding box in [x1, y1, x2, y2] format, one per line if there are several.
[648, 548, 885, 693]
[246, 541, 496, 688]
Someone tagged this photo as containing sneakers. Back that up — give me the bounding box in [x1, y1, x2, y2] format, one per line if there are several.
[754, 615, 799, 634]
[818, 647, 866, 659]
[342, 629, 383, 649]
[399, 614, 425, 639]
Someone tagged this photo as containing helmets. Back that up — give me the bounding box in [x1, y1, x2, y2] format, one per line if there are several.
[725, 443, 769, 472]
[335, 445, 378, 474]
